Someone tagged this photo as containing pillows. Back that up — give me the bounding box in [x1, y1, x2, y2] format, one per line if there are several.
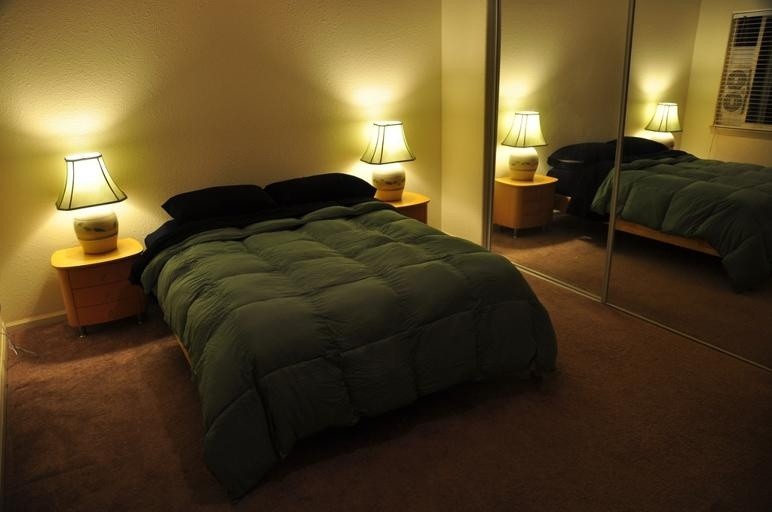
[262, 172, 378, 209]
[548, 141, 614, 170]
[607, 135, 670, 161]
[159, 181, 277, 224]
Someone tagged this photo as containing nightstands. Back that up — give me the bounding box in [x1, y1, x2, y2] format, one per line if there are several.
[372, 189, 433, 227]
[492, 174, 559, 240]
[47, 236, 149, 341]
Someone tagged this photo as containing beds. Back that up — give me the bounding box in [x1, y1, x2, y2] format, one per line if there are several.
[544, 133, 772, 296]
[130, 167, 566, 504]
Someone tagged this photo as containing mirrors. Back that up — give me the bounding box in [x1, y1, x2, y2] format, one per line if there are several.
[484, 0, 632, 306]
[600, 1, 772, 374]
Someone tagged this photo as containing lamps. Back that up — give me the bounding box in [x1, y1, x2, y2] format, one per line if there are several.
[50, 148, 132, 257]
[498, 107, 550, 182]
[642, 101, 686, 150]
[355, 117, 419, 201]
